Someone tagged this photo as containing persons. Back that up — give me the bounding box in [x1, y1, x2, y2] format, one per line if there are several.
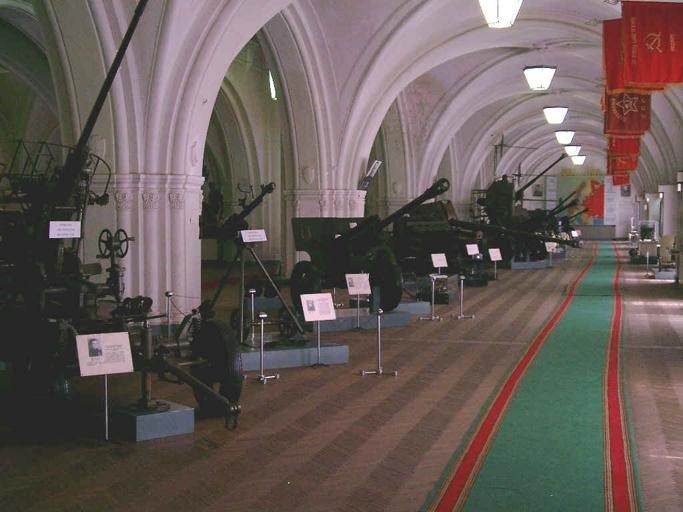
[533, 184, 542, 196]
[87, 338, 102, 357]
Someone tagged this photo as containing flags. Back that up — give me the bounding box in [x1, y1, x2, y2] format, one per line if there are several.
[599, 1, 683, 187]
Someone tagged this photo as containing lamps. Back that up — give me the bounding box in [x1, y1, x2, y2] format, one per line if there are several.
[477, 0, 523, 29]
[523, 65, 587, 166]
